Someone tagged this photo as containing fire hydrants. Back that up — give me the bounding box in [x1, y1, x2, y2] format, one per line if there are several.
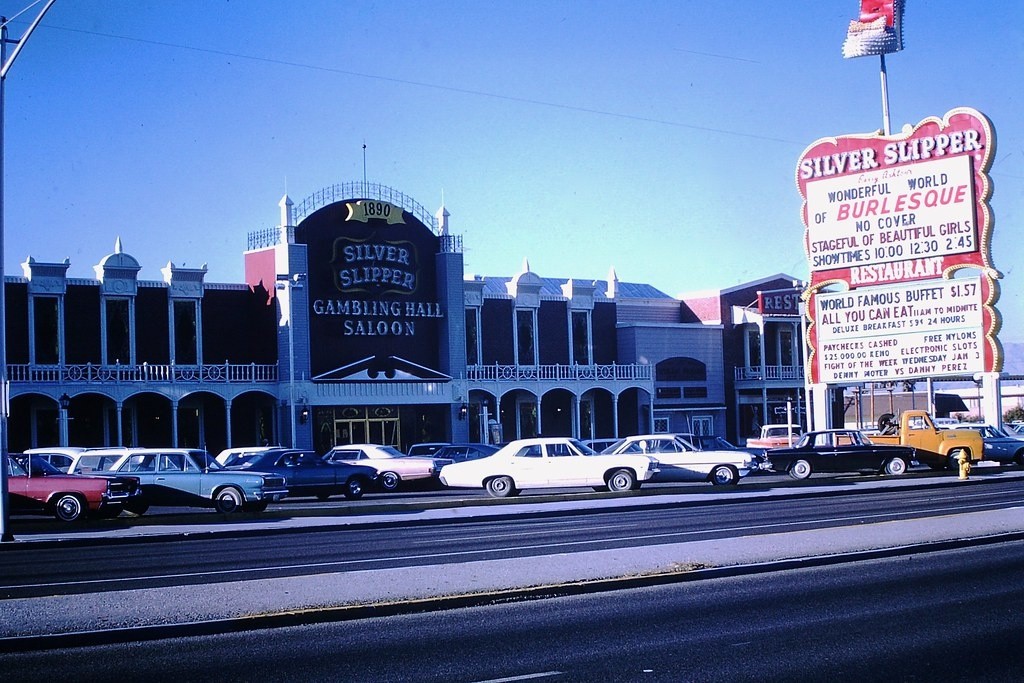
[957, 449, 972, 480]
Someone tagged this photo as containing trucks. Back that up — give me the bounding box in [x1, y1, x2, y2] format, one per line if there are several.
[745, 408, 985, 464]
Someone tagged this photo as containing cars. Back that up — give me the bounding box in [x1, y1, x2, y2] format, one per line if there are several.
[8, 452, 142, 531]
[662, 434, 774, 473]
[907, 417, 1024, 467]
[226, 449, 379, 502]
[580, 438, 649, 455]
[439, 436, 661, 498]
[67, 448, 290, 515]
[600, 433, 761, 487]
[209, 446, 288, 471]
[768, 428, 919, 480]
[407, 443, 451, 459]
[321, 444, 457, 494]
[23, 447, 129, 474]
[434, 444, 502, 480]
